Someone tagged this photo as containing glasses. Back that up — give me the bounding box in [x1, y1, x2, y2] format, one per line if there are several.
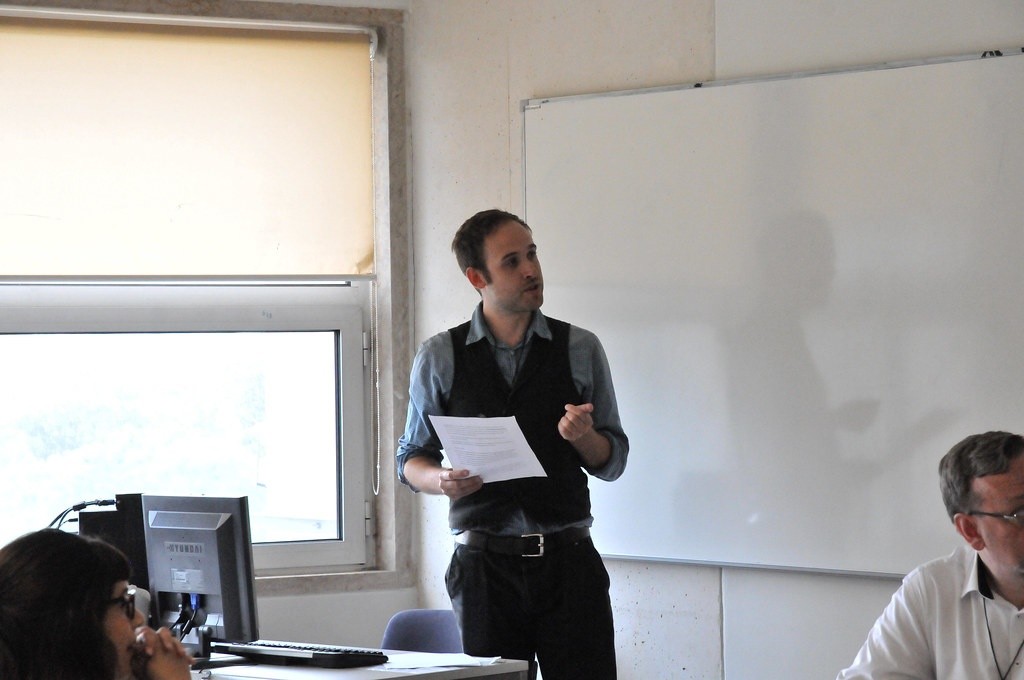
[968, 507, 1024, 526]
[107, 588, 135, 619]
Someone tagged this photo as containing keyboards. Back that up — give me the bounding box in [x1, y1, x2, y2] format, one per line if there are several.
[211, 639, 388, 668]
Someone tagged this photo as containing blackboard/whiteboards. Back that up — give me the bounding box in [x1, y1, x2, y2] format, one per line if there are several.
[522, 54, 1024, 575]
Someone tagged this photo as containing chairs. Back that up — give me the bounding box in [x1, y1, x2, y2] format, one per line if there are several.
[380, 609, 463, 653]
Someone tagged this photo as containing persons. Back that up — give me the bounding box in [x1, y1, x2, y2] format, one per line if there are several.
[396, 209, 629, 680]
[835, 431, 1024, 680]
[0, 530, 190, 680]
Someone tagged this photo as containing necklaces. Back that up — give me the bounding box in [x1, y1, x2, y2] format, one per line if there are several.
[983, 597, 1024, 680]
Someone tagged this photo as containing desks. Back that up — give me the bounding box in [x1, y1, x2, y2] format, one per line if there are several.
[188, 646, 529, 680]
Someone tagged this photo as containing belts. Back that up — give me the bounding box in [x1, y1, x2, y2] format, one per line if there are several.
[455, 526, 590, 557]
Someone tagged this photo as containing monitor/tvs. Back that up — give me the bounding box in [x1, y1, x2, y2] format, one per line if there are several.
[142, 494, 260, 671]
